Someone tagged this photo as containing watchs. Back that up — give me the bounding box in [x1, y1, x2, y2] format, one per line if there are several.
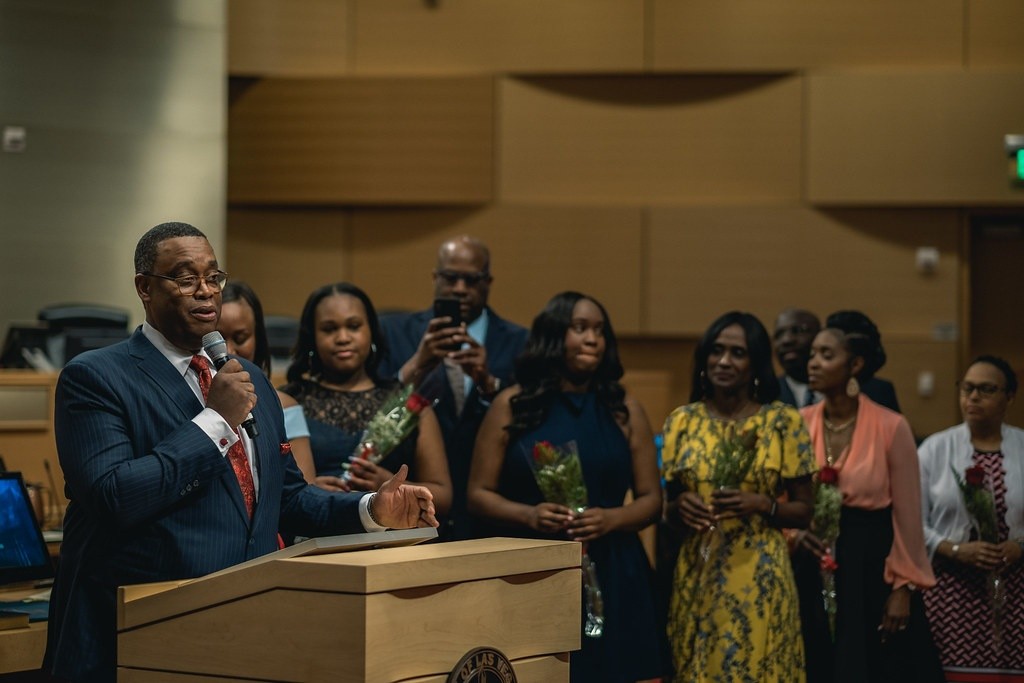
[951, 543, 960, 561]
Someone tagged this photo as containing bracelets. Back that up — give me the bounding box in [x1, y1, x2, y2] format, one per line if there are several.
[768, 494, 778, 517]
[486, 377, 500, 393]
[368, 494, 385, 526]
[900, 583, 915, 595]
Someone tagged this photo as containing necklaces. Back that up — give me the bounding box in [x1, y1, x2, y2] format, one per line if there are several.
[822, 416, 857, 467]
[712, 398, 740, 418]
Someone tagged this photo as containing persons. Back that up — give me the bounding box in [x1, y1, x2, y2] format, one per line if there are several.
[40, 221, 440, 683]
[781, 311, 945, 683]
[217, 282, 454, 515]
[373, 235, 533, 544]
[917, 353, 1024, 669]
[662, 311, 820, 683]
[762, 308, 900, 412]
[467, 289, 674, 683]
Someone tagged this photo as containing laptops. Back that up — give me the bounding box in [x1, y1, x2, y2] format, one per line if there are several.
[0, 471, 64, 583]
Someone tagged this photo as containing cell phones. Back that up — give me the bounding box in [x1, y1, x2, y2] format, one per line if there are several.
[433, 299, 462, 352]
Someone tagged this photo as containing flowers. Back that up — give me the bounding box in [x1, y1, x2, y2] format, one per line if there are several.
[950, 464, 1006, 644]
[809, 467, 843, 644]
[521, 440, 604, 636]
[294, 385, 431, 545]
[701, 426, 761, 562]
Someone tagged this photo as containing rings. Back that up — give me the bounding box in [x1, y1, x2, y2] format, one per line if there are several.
[899, 625, 906, 630]
[1003, 557, 1007, 562]
[720, 485, 725, 496]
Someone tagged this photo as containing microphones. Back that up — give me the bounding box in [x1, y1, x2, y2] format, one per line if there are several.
[202, 330, 259, 438]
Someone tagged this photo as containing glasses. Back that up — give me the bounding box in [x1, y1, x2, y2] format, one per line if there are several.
[955, 380, 1007, 399]
[137, 270, 228, 297]
[439, 272, 484, 287]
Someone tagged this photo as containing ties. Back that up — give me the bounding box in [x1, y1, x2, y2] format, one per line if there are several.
[443, 347, 465, 418]
[189, 355, 257, 523]
